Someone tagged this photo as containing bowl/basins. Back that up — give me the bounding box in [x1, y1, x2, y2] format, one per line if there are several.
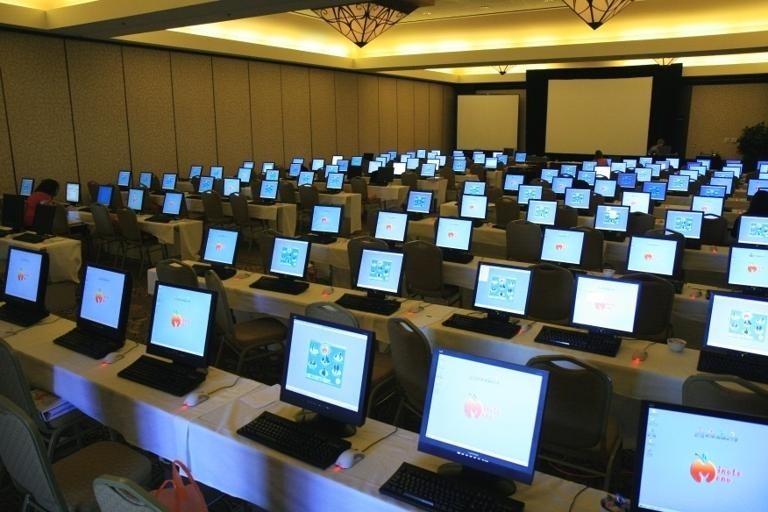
[667, 336, 686, 353]
[603, 269, 615, 277]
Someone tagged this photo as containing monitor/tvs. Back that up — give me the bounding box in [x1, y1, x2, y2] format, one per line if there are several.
[279, 311, 376, 438]
[471, 261, 534, 324]
[569, 272, 644, 339]
[76, 263, 133, 343]
[1, 196, 57, 235]
[630, 399, 768, 512]
[2, 245, 50, 312]
[355, 247, 406, 300]
[418, 346, 550, 497]
[18, 148, 768, 292]
[146, 280, 218, 368]
[700, 291, 768, 364]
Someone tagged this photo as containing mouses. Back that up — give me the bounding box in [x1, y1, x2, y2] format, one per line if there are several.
[632, 350, 647, 361]
[104, 351, 124, 364]
[335, 447, 366, 469]
[236, 271, 334, 296]
[183, 392, 210, 407]
[53, 329, 115, 361]
[412, 305, 424, 313]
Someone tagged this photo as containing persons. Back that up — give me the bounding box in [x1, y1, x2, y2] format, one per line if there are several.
[22, 178, 60, 232]
[592, 149, 608, 166]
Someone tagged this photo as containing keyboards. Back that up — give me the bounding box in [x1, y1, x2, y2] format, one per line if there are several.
[237, 410, 352, 470]
[1, 303, 47, 328]
[441, 313, 522, 339]
[335, 293, 402, 316]
[379, 461, 526, 512]
[190, 264, 310, 296]
[1, 229, 45, 244]
[695, 349, 768, 382]
[535, 326, 622, 358]
[117, 354, 206, 397]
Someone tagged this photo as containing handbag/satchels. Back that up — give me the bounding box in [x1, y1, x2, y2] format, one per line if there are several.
[149, 460, 209, 512]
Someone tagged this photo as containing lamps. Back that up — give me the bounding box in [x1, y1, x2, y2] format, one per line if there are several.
[492, 65, 514, 75]
[312, 1, 419, 48]
[563, 0, 633, 30]
[654, 57, 675, 66]
